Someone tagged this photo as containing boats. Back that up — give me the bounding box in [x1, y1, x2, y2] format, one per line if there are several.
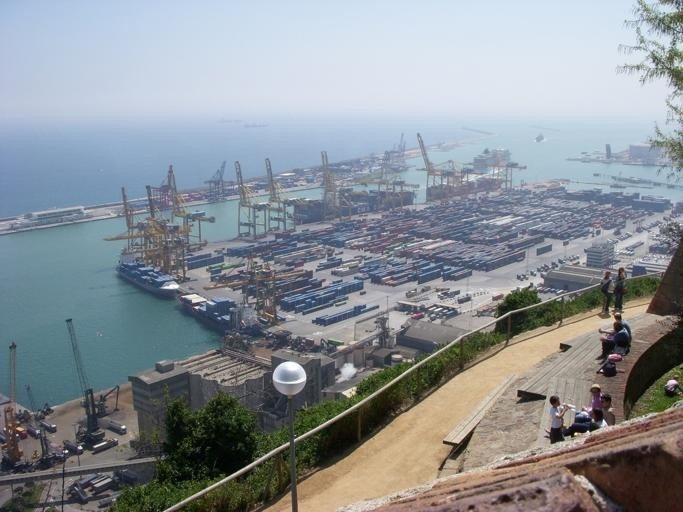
[116, 260, 178, 297]
[180, 293, 241, 331]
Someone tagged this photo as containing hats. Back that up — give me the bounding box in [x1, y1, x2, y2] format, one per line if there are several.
[590, 384, 601, 393]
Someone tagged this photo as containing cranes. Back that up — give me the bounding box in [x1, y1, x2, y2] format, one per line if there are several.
[2, 319, 119, 468]
[103, 163, 215, 282]
[232, 158, 307, 238]
[204, 249, 304, 323]
[318, 150, 406, 220]
[385, 132, 406, 163]
[416, 131, 465, 203]
[204, 161, 227, 198]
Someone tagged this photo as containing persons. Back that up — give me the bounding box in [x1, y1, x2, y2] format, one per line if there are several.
[600, 271, 616, 318]
[544, 383, 615, 444]
[596, 312, 632, 365]
[596, 354, 616, 377]
[612, 267, 625, 313]
[664, 375, 683, 396]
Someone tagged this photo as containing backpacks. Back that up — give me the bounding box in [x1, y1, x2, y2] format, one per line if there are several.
[596, 352, 622, 376]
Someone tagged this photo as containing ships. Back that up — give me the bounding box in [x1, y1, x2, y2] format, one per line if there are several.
[293, 189, 415, 224]
[425, 148, 511, 201]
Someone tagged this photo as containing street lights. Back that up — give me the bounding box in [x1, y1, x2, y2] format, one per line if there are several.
[271, 358, 307, 511]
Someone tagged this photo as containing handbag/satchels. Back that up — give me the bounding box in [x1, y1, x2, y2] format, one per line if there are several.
[614, 287, 629, 295]
[602, 280, 612, 293]
